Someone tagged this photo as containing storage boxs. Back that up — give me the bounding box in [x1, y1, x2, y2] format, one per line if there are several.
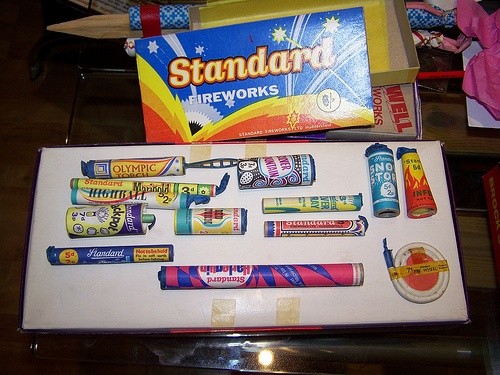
[136, 0, 421, 142]
